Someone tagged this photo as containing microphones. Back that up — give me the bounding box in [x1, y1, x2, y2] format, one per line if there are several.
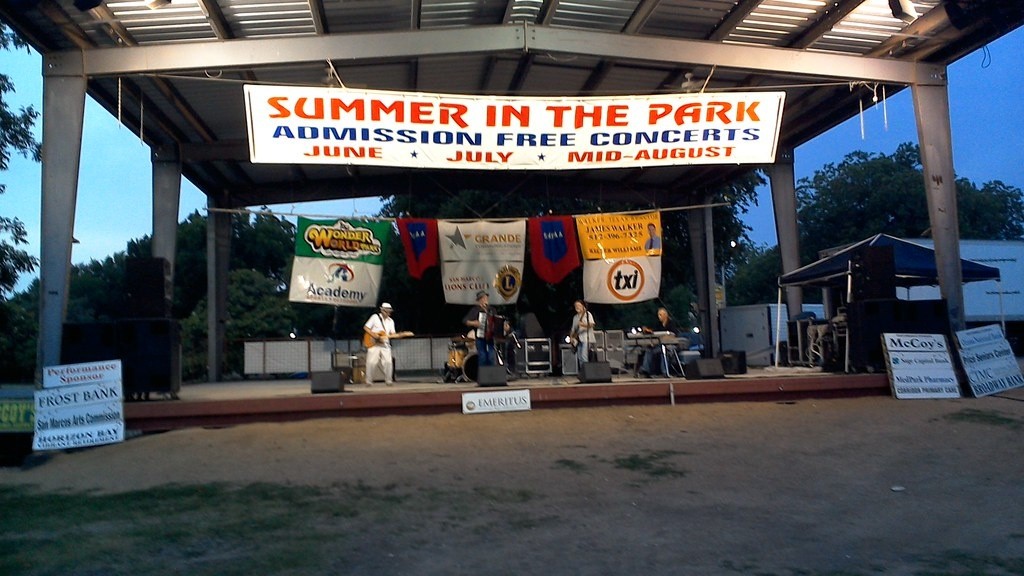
[489, 308, 494, 314]
[585, 301, 588, 309]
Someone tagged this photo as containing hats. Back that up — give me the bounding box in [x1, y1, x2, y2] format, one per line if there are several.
[379, 303, 394, 311]
[476, 291, 489, 302]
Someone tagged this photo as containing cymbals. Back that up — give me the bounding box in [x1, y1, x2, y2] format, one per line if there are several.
[451, 337, 476, 343]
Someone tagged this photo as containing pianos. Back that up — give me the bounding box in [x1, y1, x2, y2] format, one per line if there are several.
[627, 330, 680, 347]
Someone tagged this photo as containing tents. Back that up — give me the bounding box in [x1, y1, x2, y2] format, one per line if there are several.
[773, 232, 1005, 373]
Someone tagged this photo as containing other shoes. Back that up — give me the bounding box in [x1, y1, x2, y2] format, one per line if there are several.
[367, 383, 372, 386]
[387, 383, 393, 386]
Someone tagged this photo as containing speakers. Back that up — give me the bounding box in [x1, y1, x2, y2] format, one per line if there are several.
[561, 349, 578, 375]
[580, 362, 612, 383]
[134, 257, 172, 317]
[592, 331, 606, 362]
[311, 371, 345, 393]
[685, 358, 725, 379]
[605, 330, 625, 368]
[717, 351, 747, 374]
[478, 366, 506, 386]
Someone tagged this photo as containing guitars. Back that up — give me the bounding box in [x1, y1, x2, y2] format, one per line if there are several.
[364, 329, 415, 347]
[571, 307, 586, 355]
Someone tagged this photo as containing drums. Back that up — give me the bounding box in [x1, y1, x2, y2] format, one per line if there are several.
[462, 352, 479, 382]
[448, 343, 468, 368]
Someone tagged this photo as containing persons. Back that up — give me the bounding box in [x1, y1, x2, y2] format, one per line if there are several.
[644, 223, 661, 250]
[363, 303, 396, 387]
[637, 308, 677, 376]
[462, 290, 497, 366]
[569, 299, 597, 368]
